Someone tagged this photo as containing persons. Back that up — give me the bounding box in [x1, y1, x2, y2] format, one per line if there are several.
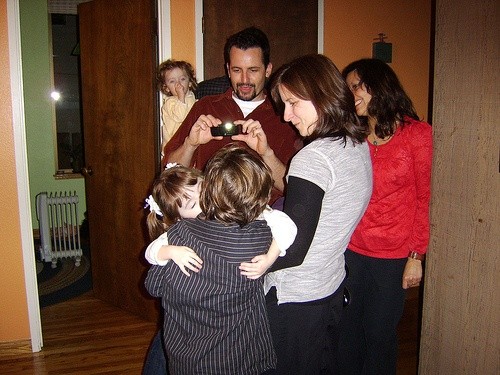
[141, 160, 297, 375]
[192, 74, 233, 99]
[161, 30, 306, 207]
[154, 59, 199, 156]
[144, 144, 280, 375]
[255, 51, 373, 374]
[334, 59, 433, 374]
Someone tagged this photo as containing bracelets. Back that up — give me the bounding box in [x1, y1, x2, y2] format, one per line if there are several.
[407, 251, 425, 262]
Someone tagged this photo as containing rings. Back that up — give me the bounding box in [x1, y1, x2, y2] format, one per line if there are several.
[412, 283, 416, 285]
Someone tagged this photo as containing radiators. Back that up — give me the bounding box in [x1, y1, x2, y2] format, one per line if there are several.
[35, 190, 83, 269]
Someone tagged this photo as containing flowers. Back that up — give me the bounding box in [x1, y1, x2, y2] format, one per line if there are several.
[164, 161, 182, 171]
[143, 195, 164, 218]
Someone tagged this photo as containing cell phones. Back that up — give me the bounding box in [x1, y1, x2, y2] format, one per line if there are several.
[210, 123, 240, 137]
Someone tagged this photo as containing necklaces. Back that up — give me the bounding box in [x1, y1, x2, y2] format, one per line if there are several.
[371, 130, 380, 146]
